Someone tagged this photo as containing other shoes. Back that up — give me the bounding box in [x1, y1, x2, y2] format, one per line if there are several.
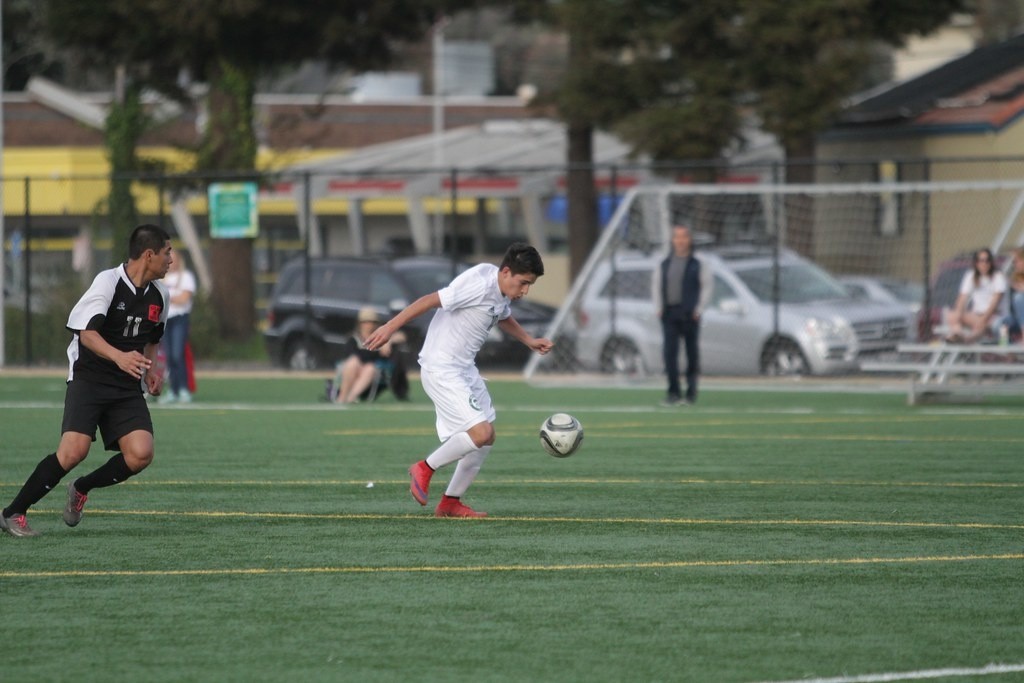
[178, 388, 191, 403]
[157, 389, 178, 403]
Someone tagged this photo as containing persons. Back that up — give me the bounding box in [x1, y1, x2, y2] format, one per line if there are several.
[362, 242, 556, 519]
[156, 252, 198, 404]
[945, 248, 1012, 343]
[992, 248, 1024, 341]
[337, 304, 407, 404]
[651, 226, 712, 407]
[0, 224, 173, 538]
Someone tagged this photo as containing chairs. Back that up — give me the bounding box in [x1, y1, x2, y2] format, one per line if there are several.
[330, 343, 399, 405]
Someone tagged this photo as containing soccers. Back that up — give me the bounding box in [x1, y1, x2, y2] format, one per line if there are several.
[539, 413, 585, 459]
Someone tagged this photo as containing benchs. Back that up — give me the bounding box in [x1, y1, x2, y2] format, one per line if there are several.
[857, 316, 1024, 406]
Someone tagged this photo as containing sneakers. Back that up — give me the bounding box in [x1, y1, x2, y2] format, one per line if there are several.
[408, 459, 434, 505]
[63, 476, 88, 527]
[435, 493, 487, 518]
[0, 507, 40, 538]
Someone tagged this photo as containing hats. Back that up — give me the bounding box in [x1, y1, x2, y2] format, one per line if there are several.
[358, 306, 378, 321]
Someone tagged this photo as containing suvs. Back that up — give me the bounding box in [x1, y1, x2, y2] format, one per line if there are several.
[261, 251, 559, 375]
[574, 244, 918, 381]
[918, 253, 1018, 336]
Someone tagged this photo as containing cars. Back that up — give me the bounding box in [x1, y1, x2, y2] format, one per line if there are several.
[797, 276, 927, 321]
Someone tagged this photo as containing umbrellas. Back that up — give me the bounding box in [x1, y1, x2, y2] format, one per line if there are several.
[820, 34, 1024, 144]
